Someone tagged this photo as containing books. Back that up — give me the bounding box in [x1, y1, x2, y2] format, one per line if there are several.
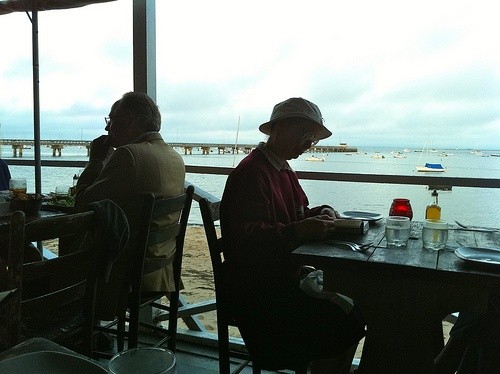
[332, 218, 370, 235]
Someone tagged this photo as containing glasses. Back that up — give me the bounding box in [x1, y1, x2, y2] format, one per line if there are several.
[297, 123, 319, 148]
[105, 114, 127, 124]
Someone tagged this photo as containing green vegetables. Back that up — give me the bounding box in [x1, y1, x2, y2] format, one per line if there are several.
[64, 195, 74, 207]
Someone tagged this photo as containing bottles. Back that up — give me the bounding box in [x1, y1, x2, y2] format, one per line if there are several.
[68, 174, 80, 207]
[426, 193, 442, 221]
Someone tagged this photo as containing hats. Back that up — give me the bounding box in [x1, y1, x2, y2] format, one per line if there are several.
[81, 199, 131, 283]
[259, 98, 332, 141]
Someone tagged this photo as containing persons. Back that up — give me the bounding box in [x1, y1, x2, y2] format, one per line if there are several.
[21, 91, 185, 354]
[0, 159, 42, 292]
[434, 278, 500, 374]
[220, 98, 360, 374]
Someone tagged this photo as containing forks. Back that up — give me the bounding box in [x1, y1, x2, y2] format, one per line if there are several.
[454, 220, 500, 230]
[329, 238, 373, 251]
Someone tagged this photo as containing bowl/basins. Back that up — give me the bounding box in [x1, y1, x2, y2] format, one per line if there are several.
[14, 194, 43, 216]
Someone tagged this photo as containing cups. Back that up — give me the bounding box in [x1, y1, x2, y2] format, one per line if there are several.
[0, 200, 11, 217]
[9, 178, 27, 194]
[422, 219, 449, 251]
[0, 191, 14, 201]
[388, 198, 413, 220]
[385, 216, 411, 246]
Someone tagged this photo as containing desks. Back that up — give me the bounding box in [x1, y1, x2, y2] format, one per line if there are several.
[0, 195, 86, 261]
[291, 210, 500, 374]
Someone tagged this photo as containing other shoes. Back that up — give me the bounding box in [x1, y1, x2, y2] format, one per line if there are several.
[53, 322, 114, 352]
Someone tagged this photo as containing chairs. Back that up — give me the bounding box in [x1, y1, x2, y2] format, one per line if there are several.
[0, 184, 362, 374]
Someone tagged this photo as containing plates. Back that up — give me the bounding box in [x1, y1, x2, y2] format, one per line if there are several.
[43, 192, 68, 210]
[454, 246, 500, 266]
[343, 211, 383, 224]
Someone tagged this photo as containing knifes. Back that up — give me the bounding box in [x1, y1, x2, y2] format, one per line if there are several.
[356, 242, 387, 248]
